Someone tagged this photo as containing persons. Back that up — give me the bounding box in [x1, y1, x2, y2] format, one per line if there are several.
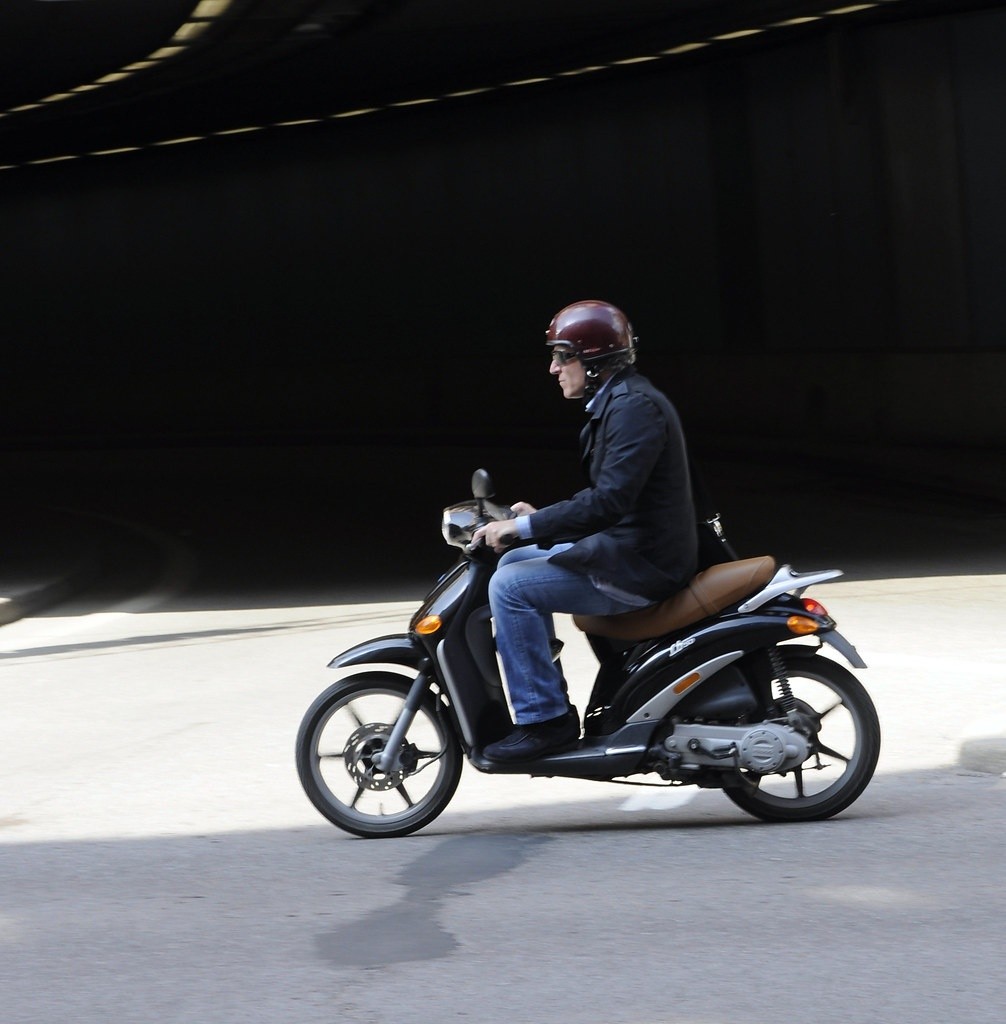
[469, 298, 698, 767]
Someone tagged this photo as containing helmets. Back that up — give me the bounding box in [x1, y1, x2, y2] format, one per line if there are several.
[546, 299, 637, 372]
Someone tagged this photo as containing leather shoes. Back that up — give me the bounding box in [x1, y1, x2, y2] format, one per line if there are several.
[485, 705, 581, 762]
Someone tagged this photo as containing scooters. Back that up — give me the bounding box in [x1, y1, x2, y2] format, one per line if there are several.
[293, 467, 883, 838]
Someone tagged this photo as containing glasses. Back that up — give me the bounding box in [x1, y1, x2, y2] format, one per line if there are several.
[551, 350, 577, 367]
